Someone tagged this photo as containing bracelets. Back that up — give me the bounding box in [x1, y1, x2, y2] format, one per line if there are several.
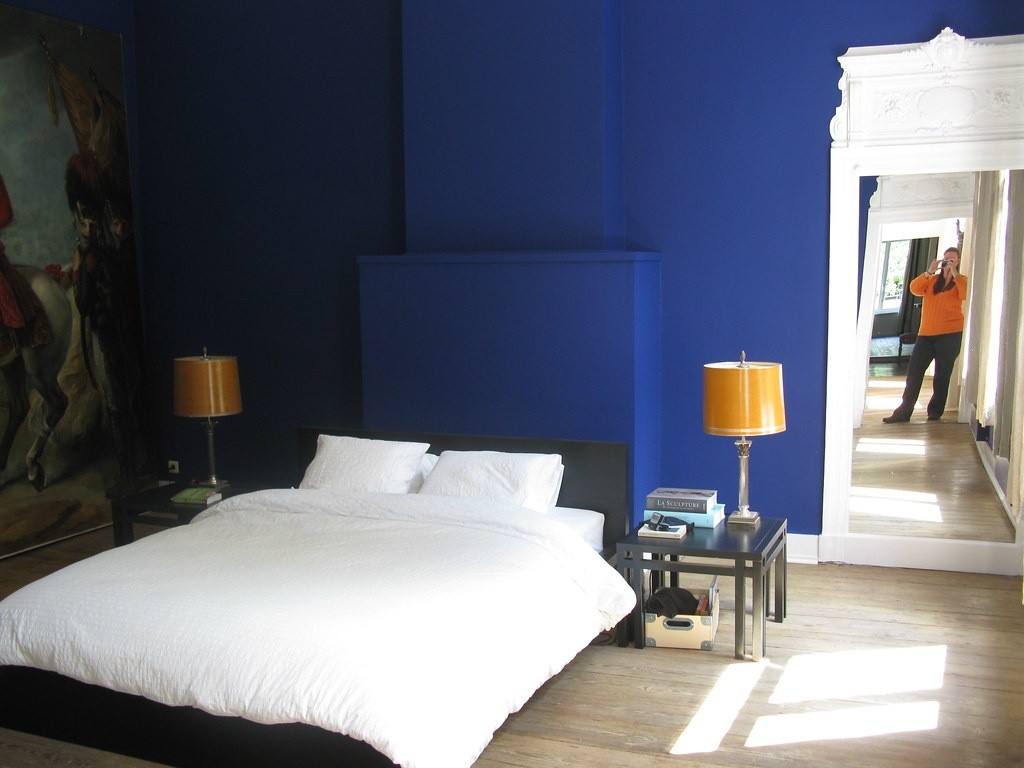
[927, 270, 932, 275]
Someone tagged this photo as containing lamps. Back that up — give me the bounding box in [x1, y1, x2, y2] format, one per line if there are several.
[172, 346, 243, 487]
[702, 351, 786, 524]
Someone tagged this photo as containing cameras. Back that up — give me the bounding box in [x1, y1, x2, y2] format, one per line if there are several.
[942, 260, 953, 267]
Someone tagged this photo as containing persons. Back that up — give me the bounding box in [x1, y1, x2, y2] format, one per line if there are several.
[883, 247, 967, 423]
[657, 490, 714, 496]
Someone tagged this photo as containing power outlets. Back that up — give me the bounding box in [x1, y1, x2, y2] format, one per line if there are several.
[168, 461, 179, 474]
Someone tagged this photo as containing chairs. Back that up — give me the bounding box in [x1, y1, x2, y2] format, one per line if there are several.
[897, 302, 922, 366]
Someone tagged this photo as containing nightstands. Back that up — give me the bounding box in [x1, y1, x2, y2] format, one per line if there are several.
[111, 482, 242, 547]
[616, 514, 788, 662]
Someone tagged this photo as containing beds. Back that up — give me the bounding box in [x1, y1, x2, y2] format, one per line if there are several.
[0, 434, 630, 768]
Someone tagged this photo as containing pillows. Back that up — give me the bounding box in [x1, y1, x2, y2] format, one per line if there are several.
[300, 434, 431, 494]
[418, 450, 562, 511]
[408, 453, 439, 494]
[548, 464, 565, 510]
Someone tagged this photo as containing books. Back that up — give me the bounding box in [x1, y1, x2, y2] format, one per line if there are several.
[695, 575, 718, 615]
[173, 488, 222, 504]
[644, 488, 725, 528]
[638, 524, 686, 538]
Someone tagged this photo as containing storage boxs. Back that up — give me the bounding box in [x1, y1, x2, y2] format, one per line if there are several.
[645, 587, 720, 651]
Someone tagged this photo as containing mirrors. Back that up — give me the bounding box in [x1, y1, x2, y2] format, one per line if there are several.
[820, 26, 1024, 577]
